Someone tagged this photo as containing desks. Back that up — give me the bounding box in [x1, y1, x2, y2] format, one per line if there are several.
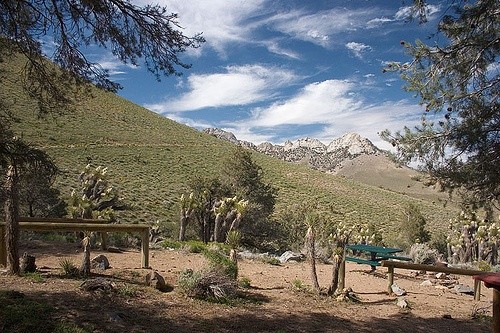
[346, 243, 403, 255]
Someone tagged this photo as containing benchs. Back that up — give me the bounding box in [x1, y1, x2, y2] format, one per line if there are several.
[366, 253, 413, 262]
[345, 256, 379, 268]
